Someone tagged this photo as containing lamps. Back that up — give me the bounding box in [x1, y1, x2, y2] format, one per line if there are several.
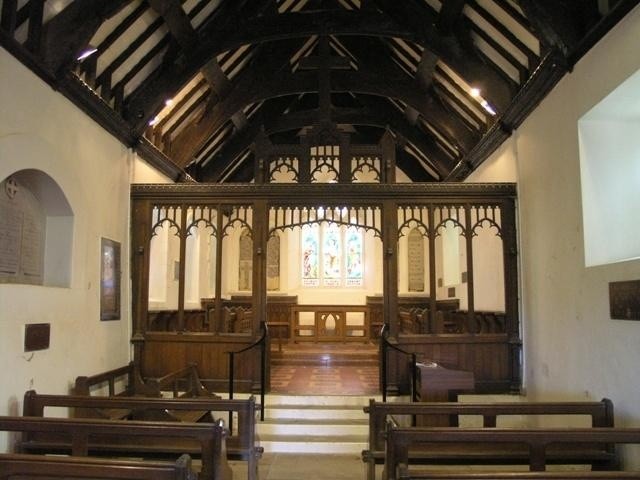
[77, 44, 98, 61]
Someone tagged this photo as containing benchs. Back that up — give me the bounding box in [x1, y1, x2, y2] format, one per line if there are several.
[392, 304, 508, 338]
[0, 359, 266, 480]
[153, 303, 269, 336]
[358, 394, 640, 480]
